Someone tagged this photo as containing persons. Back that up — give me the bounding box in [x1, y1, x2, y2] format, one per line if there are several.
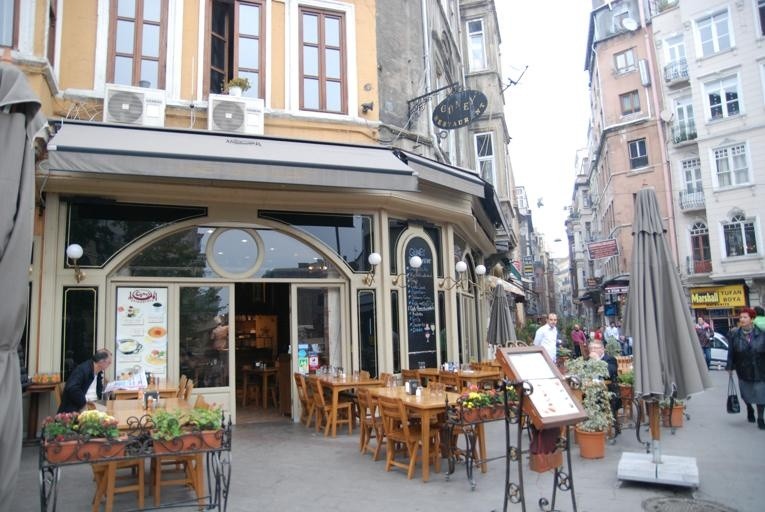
[750, 306, 765, 333]
[532, 312, 558, 364]
[725, 308, 765, 430]
[695, 313, 715, 368]
[56, 348, 113, 413]
[180, 332, 200, 369]
[557, 316, 626, 358]
[588, 340, 623, 439]
[209, 313, 229, 350]
[731, 321, 741, 332]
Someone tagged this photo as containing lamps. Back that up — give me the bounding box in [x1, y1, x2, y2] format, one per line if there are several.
[460, 264, 486, 296]
[439, 261, 468, 291]
[363, 253, 381, 287]
[67, 244, 88, 282]
[391, 255, 422, 289]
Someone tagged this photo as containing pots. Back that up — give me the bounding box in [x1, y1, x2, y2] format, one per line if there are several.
[116, 339, 142, 354]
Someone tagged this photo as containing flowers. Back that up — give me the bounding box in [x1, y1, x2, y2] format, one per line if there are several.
[145, 403, 226, 441]
[455, 384, 519, 408]
[42, 410, 125, 443]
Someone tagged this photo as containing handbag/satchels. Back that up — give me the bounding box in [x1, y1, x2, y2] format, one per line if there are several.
[727, 395, 740, 412]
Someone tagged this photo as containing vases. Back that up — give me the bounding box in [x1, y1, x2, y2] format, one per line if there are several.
[48, 433, 128, 463]
[150, 429, 222, 453]
[461, 401, 515, 424]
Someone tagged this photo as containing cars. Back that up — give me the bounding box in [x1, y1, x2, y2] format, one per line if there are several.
[711, 332, 729, 370]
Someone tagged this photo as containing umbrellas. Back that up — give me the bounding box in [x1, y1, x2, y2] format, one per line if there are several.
[0, 63, 43, 511]
[486, 283, 519, 347]
[617, 186, 713, 488]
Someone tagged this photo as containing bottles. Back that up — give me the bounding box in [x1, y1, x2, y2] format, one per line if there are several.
[148, 395, 158, 412]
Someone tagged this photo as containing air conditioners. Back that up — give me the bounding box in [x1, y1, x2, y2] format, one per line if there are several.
[102, 83, 167, 127]
[208, 92, 265, 136]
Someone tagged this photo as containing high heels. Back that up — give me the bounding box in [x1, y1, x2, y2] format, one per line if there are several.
[748, 411, 755, 423]
[757, 419, 765, 429]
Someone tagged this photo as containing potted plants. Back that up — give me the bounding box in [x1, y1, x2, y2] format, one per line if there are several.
[227, 77, 251, 96]
[659, 398, 688, 427]
[565, 356, 616, 459]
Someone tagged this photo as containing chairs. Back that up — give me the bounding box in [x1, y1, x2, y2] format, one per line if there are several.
[294, 361, 505, 481]
[54, 375, 215, 510]
[234, 362, 288, 409]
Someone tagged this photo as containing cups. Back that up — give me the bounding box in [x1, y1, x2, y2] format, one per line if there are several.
[316, 362, 470, 397]
